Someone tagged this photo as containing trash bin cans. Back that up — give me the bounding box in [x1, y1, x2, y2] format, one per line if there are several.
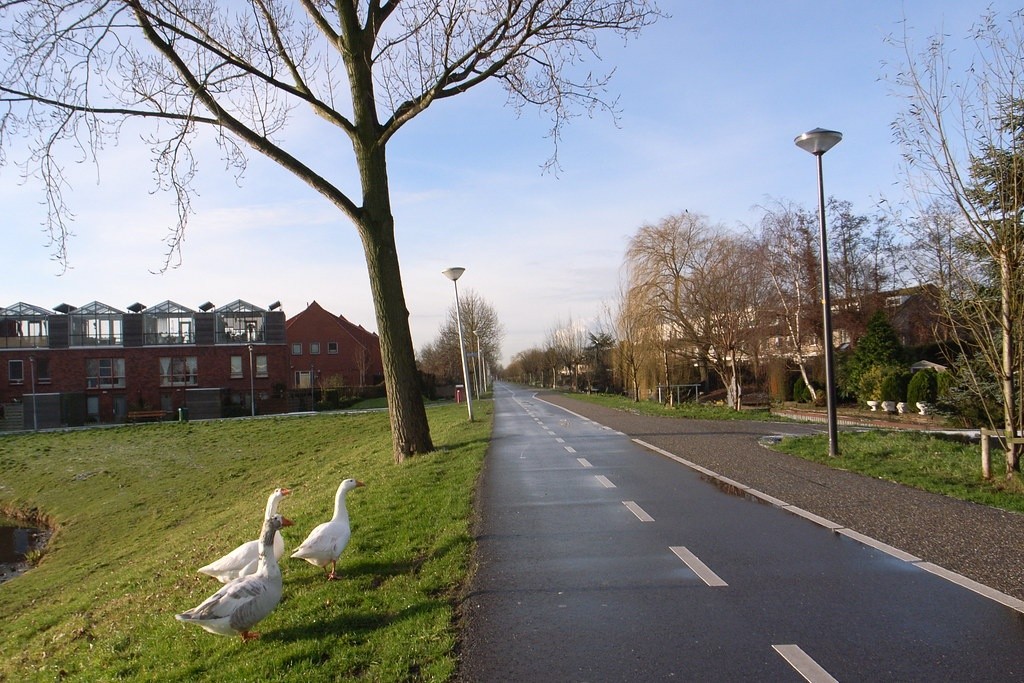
[178, 408, 189, 423]
[455, 385, 465, 403]
[670, 391, 683, 407]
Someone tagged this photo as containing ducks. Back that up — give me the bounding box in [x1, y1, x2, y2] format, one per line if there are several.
[196, 489, 293, 583]
[174, 514, 296, 645]
[290, 479, 366, 581]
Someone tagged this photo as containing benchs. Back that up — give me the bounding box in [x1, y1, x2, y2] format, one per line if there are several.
[128, 409, 168, 425]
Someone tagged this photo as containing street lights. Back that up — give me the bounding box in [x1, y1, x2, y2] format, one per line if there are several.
[793, 127, 843, 459]
[442, 266, 475, 423]
[472, 330, 483, 399]
